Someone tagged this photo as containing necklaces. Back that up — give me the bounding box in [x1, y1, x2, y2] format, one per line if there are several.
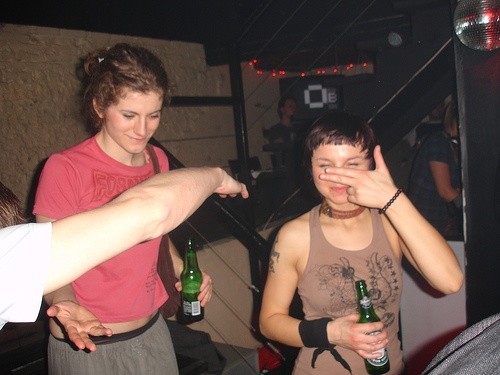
[319, 198, 367, 220]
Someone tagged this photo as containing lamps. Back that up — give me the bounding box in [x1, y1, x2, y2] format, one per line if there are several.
[387, 26, 413, 47]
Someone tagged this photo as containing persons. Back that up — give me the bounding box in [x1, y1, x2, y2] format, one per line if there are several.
[0, 165, 250, 333]
[32, 42, 215, 375]
[405, 97, 463, 242]
[258, 111, 462, 374]
[269, 96, 299, 166]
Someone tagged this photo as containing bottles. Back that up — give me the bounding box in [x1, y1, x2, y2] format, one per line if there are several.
[355, 279, 390, 375]
[180, 238, 204, 323]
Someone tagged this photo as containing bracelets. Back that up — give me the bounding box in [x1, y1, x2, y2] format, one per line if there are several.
[298, 317, 336, 349]
[379, 187, 404, 216]
